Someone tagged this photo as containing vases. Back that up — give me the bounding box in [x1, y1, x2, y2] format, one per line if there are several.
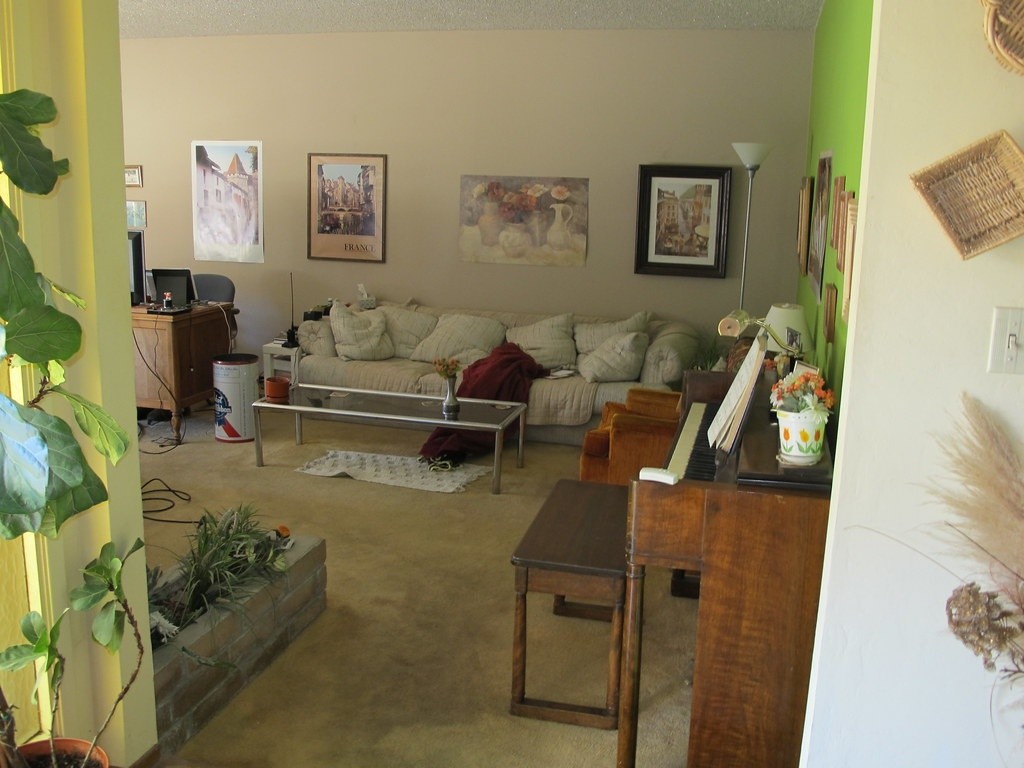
[148, 529, 327, 759]
[443, 376, 459, 418]
[775, 410, 826, 465]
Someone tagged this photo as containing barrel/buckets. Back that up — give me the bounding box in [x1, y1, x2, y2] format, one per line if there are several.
[212, 353, 259, 442]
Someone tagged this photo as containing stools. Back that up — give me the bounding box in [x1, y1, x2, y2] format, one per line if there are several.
[510, 479, 628, 731]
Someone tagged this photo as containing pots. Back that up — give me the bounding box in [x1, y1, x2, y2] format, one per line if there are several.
[266, 376, 290, 397]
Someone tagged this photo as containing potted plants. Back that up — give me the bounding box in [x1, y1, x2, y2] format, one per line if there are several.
[0, 90, 144, 768]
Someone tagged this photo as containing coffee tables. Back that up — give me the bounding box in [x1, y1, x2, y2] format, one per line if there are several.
[252, 383, 526, 494]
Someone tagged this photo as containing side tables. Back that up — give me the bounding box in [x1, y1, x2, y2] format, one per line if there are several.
[263, 337, 302, 396]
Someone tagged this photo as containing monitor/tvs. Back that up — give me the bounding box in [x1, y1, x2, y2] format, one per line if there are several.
[127, 230, 147, 306]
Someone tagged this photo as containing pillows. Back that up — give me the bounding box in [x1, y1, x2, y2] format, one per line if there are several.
[578, 333, 648, 382]
[506, 311, 577, 369]
[574, 310, 649, 371]
[375, 305, 438, 359]
[329, 300, 394, 361]
[410, 313, 507, 363]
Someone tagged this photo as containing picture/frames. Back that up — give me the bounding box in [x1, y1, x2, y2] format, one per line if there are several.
[125, 200, 146, 228]
[307, 153, 386, 263]
[797, 177, 811, 276]
[634, 164, 732, 280]
[125, 165, 142, 187]
[793, 360, 820, 376]
[837, 191, 853, 274]
[822, 284, 836, 342]
[830, 176, 845, 249]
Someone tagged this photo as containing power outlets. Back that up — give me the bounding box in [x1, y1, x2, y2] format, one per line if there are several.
[986, 307, 1024, 375]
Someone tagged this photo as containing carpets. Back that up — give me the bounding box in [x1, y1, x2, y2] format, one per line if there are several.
[293, 450, 494, 493]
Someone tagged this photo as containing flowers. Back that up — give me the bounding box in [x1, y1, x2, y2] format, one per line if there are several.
[143, 503, 293, 675]
[434, 352, 465, 379]
[770, 371, 834, 424]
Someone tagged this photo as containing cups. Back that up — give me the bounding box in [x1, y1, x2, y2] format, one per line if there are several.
[163, 299, 172, 308]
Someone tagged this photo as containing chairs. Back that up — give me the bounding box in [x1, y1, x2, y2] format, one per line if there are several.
[191, 274, 239, 339]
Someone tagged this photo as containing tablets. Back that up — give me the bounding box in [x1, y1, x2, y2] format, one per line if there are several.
[151, 268, 197, 305]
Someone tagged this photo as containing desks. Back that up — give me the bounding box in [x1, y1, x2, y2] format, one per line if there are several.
[131, 302, 234, 443]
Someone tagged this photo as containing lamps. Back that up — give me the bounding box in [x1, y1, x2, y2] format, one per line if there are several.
[730, 142, 774, 179]
[718, 310, 809, 379]
[752, 303, 814, 378]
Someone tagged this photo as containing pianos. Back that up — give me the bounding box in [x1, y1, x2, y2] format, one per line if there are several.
[617, 369, 833, 768]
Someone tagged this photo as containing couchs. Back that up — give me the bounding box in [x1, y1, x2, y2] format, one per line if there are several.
[295, 299, 699, 446]
[578, 387, 684, 486]
